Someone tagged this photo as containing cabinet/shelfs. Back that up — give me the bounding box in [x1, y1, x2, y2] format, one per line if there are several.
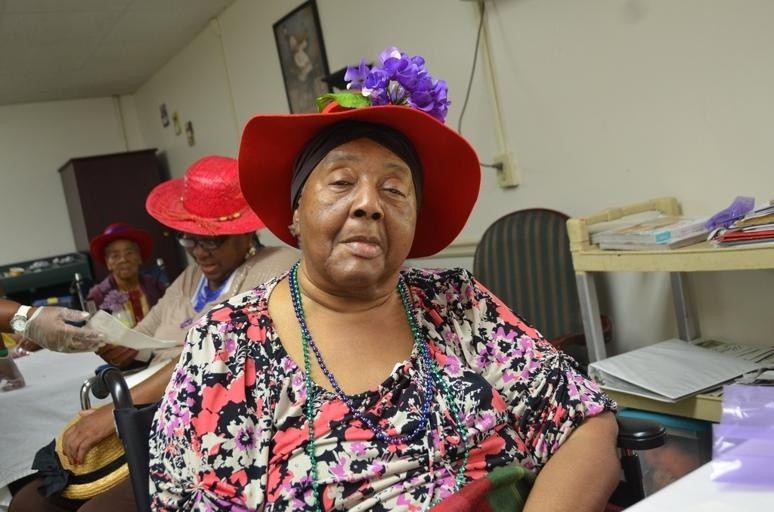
[0, 251, 96, 310]
[54, 149, 180, 281]
[563, 190, 772, 432]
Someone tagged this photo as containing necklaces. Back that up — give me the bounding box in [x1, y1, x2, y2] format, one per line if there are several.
[289, 261, 468, 511]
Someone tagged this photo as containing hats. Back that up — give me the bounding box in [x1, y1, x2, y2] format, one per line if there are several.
[55, 405, 130, 500]
[90, 221, 154, 267]
[145, 156, 267, 237]
[238, 89, 481, 259]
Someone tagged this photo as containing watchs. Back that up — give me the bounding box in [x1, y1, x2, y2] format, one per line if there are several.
[9, 302, 34, 337]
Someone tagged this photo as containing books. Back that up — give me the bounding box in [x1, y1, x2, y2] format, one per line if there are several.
[587, 209, 713, 252]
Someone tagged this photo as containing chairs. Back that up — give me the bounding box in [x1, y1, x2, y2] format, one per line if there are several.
[86, 361, 666, 512]
[469, 205, 611, 374]
[70, 255, 171, 321]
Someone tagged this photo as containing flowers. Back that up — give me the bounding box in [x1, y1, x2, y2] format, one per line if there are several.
[307, 39, 453, 130]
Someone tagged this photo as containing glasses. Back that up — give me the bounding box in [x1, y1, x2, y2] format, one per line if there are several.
[176, 233, 228, 250]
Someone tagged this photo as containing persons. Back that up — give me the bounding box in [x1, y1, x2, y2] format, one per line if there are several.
[6, 157, 302, 510]
[83, 216, 165, 329]
[0, 297, 108, 355]
[145, 46, 620, 512]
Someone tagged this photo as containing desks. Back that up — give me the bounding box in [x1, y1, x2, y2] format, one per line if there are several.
[0, 337, 170, 508]
[616, 441, 774, 512]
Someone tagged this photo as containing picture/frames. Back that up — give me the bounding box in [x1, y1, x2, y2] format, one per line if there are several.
[269, 0, 334, 118]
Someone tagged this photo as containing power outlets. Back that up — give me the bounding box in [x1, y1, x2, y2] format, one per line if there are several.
[489, 155, 520, 192]
